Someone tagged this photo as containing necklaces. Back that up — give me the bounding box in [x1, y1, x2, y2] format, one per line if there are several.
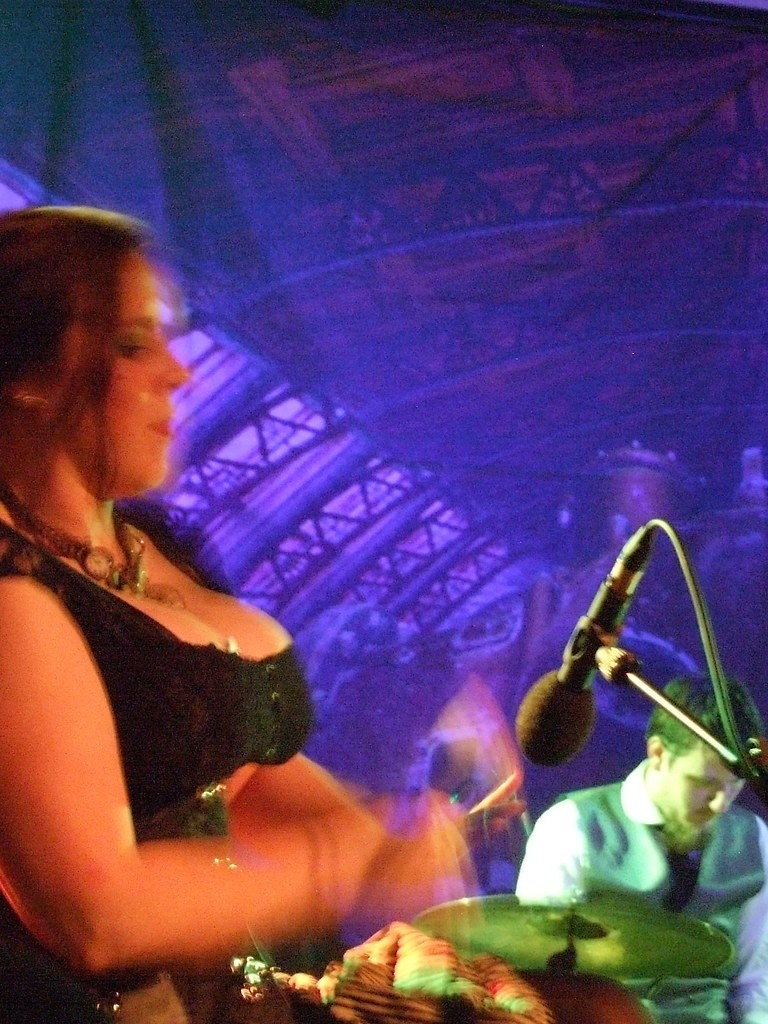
[0, 480, 183, 608]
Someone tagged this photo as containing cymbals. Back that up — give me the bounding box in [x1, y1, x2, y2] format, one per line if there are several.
[413, 892, 735, 985]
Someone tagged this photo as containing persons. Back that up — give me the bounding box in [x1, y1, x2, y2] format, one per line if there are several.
[0, 206, 528, 1024]
[516, 674, 768, 1024]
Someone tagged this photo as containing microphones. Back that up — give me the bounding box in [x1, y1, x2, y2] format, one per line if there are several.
[515, 525, 662, 768]
